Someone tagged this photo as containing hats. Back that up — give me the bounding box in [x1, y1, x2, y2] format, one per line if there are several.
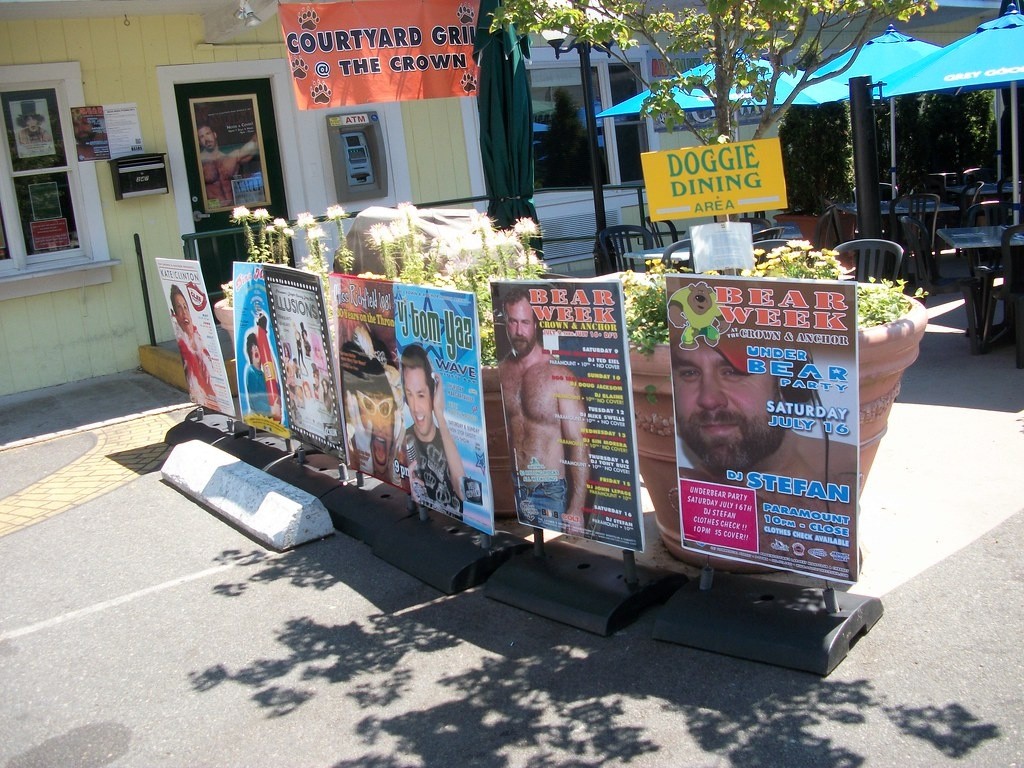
[16, 100, 44, 128]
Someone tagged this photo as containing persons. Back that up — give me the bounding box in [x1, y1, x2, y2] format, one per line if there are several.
[671, 330, 857, 584]
[280, 319, 334, 420]
[15, 109, 54, 146]
[196, 122, 259, 208]
[166, 284, 222, 411]
[341, 339, 402, 493]
[402, 344, 467, 526]
[498, 289, 594, 538]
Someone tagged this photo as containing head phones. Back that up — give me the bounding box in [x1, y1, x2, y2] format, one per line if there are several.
[776, 351, 821, 407]
[400, 342, 438, 394]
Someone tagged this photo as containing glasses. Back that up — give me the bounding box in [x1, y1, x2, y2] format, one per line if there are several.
[355, 390, 395, 418]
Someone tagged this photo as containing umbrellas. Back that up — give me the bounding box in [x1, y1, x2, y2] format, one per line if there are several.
[594, 48, 851, 143]
[810, 24, 944, 203]
[874, 3, 1024, 227]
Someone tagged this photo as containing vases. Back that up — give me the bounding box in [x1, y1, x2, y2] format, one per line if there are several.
[627, 293, 929, 573]
[214, 298, 235, 349]
[481, 367, 519, 518]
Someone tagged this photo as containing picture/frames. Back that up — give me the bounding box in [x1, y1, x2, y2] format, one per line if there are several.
[189, 93, 271, 215]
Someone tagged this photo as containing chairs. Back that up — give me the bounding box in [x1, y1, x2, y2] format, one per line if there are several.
[599, 166, 1024, 368]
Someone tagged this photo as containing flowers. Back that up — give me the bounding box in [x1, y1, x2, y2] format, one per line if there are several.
[618, 239, 929, 357]
[220, 204, 356, 308]
[357, 201, 552, 369]
